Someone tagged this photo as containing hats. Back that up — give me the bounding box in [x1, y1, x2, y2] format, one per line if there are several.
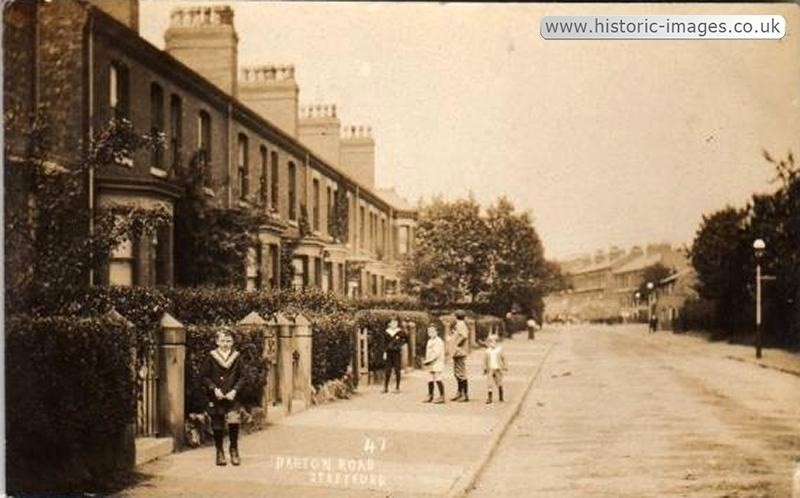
[455, 309, 467, 317]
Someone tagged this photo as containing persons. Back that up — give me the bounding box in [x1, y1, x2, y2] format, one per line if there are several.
[422, 320, 450, 404]
[378, 313, 408, 395]
[479, 331, 510, 405]
[197, 326, 249, 468]
[443, 308, 475, 403]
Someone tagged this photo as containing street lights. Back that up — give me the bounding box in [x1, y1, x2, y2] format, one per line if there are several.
[753, 239, 765, 360]
[635, 292, 640, 318]
[646, 282, 653, 321]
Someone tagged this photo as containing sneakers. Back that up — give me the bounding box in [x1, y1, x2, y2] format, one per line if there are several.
[421, 393, 507, 406]
[215, 448, 241, 467]
